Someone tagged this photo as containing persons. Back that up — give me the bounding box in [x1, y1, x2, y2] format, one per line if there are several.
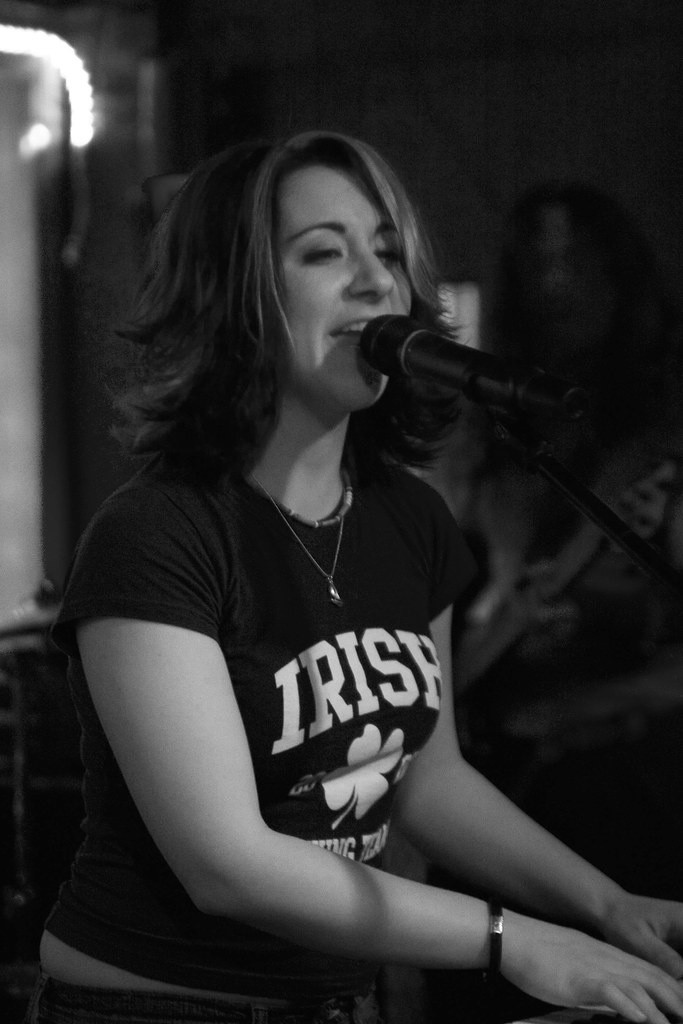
[28, 125, 683, 1023]
[421, 180, 683, 908]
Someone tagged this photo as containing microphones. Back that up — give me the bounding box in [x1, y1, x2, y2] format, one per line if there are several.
[360, 313, 590, 422]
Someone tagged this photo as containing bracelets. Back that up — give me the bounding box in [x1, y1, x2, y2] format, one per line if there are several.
[481, 895, 506, 990]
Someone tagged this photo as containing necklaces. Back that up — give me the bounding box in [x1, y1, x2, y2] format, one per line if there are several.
[252, 471, 349, 607]
[246, 471, 353, 527]
